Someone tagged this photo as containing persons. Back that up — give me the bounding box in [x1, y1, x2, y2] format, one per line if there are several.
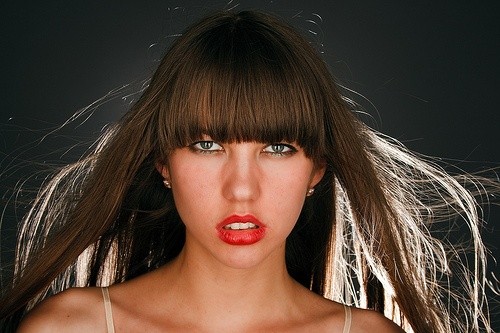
[0, 11, 500, 332]
